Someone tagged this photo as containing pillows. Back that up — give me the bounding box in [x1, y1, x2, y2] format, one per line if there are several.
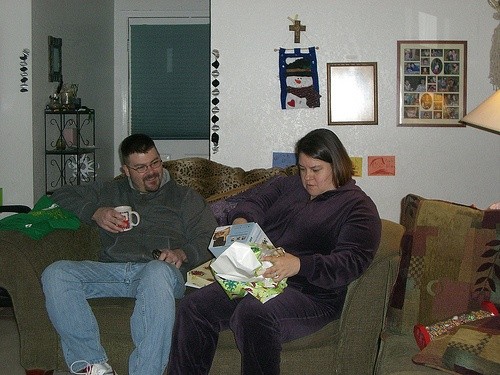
[411, 316, 500, 375]
[380, 193, 500, 326]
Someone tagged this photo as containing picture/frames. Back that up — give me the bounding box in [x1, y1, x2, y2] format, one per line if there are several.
[396, 41, 467, 127]
[327, 62, 378, 125]
[48, 36, 63, 82]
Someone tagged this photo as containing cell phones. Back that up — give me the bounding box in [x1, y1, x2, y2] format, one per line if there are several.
[153, 249, 162, 260]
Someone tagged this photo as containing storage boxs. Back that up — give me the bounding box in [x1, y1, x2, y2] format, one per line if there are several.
[208, 222, 275, 259]
[186, 261, 216, 287]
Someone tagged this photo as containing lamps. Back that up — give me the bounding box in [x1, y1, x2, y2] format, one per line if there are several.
[458, 88, 500, 135]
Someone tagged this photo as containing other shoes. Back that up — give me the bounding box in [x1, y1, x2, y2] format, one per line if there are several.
[69, 360, 119, 375]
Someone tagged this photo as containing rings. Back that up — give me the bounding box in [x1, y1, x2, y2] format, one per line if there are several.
[274, 273, 278, 276]
[171, 261, 176, 264]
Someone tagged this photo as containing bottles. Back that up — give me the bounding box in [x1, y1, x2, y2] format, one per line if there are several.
[56, 139, 66, 149]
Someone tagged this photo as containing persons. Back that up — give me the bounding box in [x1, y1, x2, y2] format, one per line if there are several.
[404, 49, 458, 118]
[41, 134, 218, 375]
[168, 128, 382, 375]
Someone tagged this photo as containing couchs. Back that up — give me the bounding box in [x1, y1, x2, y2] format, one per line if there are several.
[0, 158, 500, 375]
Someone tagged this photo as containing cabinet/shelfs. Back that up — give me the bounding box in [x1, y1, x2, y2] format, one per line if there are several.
[44, 108, 95, 198]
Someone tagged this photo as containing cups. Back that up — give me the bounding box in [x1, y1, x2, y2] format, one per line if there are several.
[114, 206, 140, 232]
[49, 91, 81, 109]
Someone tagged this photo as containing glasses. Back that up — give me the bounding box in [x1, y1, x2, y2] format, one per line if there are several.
[123, 158, 162, 173]
